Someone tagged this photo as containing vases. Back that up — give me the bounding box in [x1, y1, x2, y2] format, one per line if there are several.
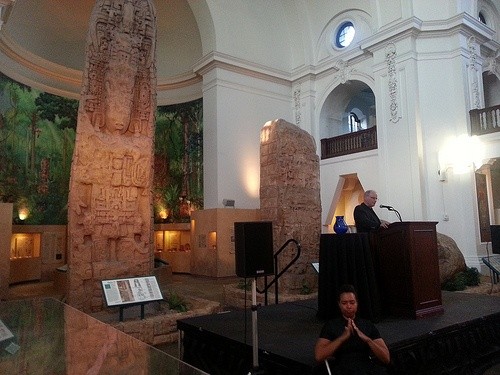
[333, 216, 348, 234]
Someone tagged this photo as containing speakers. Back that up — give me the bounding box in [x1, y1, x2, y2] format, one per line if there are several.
[232, 222, 274, 277]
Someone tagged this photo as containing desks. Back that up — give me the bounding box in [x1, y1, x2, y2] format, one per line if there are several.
[319, 234, 370, 317]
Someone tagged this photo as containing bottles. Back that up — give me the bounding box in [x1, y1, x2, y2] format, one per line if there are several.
[333, 216, 348, 233]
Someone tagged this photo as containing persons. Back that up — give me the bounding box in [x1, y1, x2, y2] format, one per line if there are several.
[354, 190, 389, 233]
[314, 285, 390, 375]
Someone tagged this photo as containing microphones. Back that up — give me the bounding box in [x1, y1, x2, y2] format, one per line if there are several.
[380, 204, 394, 208]
